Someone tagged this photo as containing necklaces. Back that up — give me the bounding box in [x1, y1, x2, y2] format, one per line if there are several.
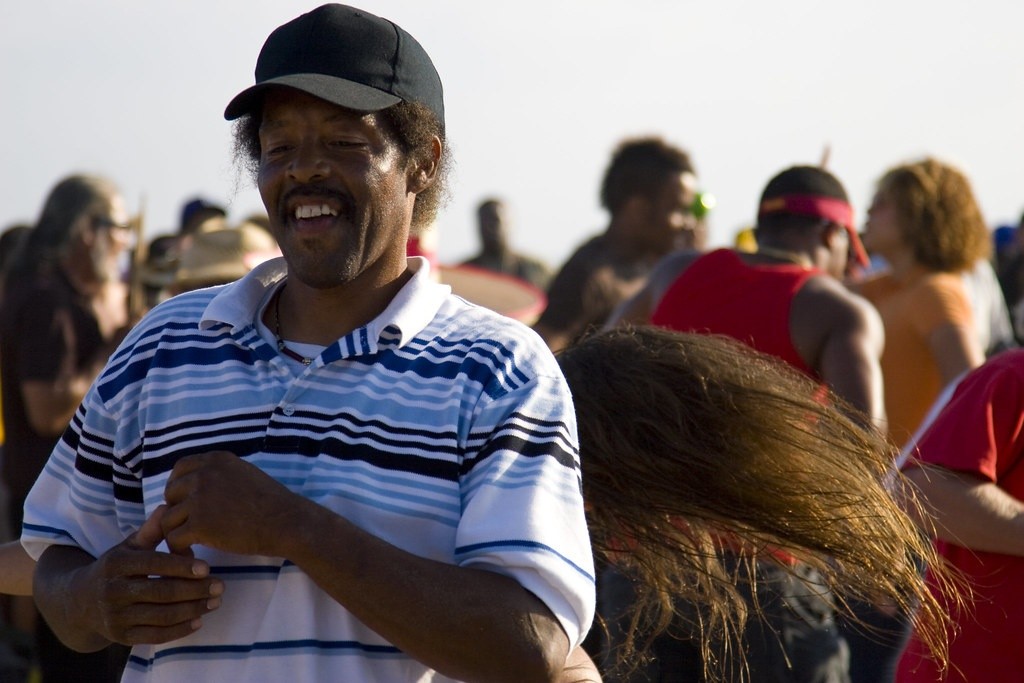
[273, 287, 317, 366]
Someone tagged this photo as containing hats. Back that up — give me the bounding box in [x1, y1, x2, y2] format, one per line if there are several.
[758, 195, 871, 270]
[177, 220, 276, 283]
[224, 3, 444, 135]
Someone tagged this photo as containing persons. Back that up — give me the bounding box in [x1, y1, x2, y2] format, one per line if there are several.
[0, 139, 1024, 682]
[21, 3, 598, 683]
